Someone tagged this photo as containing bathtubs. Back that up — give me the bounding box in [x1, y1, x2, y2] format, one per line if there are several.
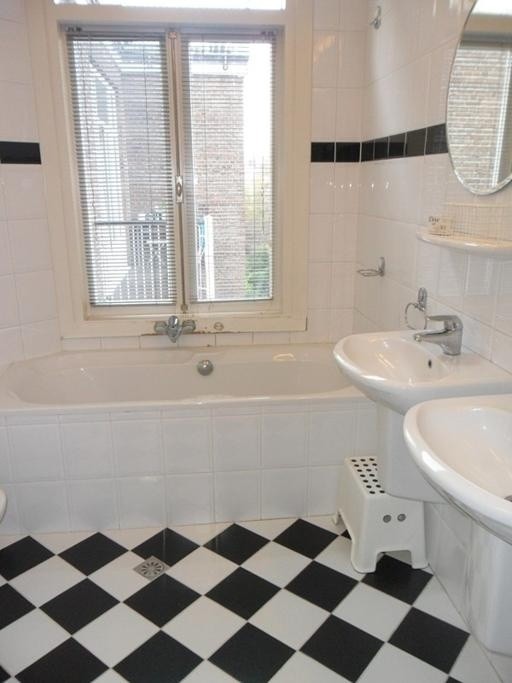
[0, 344, 378, 534]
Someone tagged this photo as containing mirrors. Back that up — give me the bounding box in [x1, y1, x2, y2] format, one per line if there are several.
[444, 0, 512, 195]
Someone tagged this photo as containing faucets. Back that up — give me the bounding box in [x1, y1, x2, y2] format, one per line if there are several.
[414, 312, 462, 358]
[167, 316, 180, 342]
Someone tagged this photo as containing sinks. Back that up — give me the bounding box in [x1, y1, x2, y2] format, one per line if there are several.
[334, 327, 512, 407]
[403, 394, 511, 541]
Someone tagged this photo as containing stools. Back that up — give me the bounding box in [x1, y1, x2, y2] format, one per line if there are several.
[333, 455, 429, 573]
